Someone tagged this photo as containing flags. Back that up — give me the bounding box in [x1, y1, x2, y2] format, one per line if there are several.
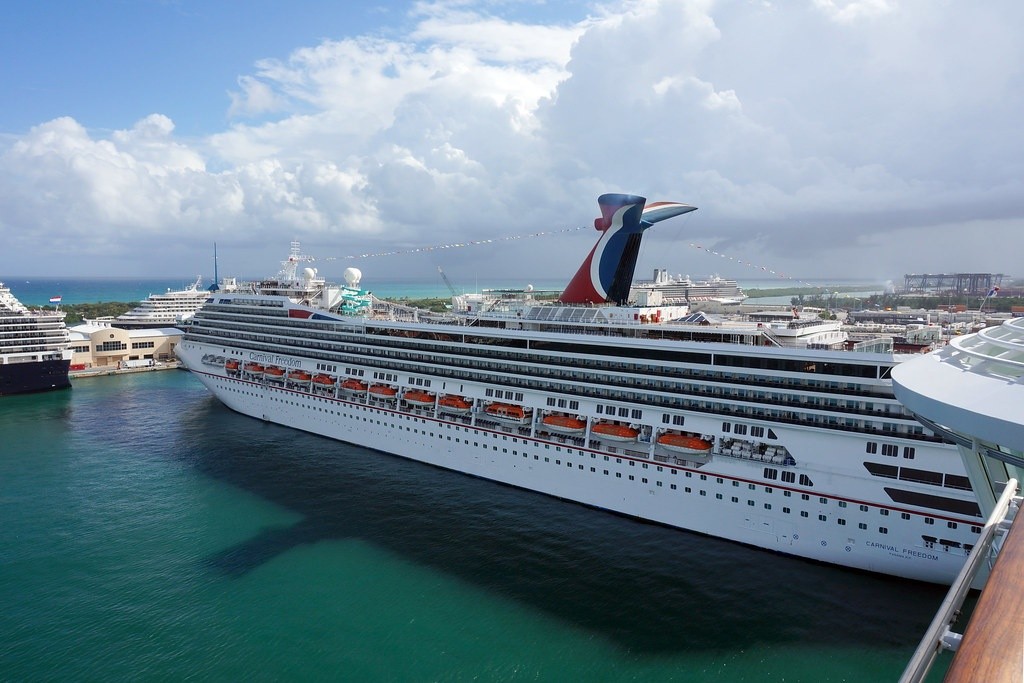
[988, 290, 997, 297]
[993, 287, 999, 291]
[49, 295, 61, 303]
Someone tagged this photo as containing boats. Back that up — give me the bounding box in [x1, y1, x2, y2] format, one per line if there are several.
[110, 277, 211, 332]
[166, 194, 1023, 591]
[0, 282, 72, 397]
[84, 316, 113, 325]
[629, 268, 749, 304]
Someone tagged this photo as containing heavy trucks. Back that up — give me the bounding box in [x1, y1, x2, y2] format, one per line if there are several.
[123, 359, 161, 369]
